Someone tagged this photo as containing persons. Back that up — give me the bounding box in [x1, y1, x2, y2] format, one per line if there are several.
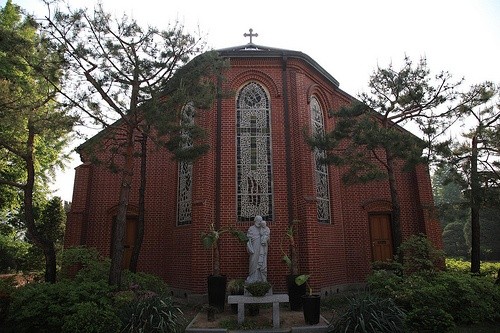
[259, 220, 270, 245]
[247, 216, 270, 282]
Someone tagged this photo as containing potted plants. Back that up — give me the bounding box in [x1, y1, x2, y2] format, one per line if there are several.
[281, 220, 306, 312]
[296, 274, 321, 325]
[201, 227, 248, 313]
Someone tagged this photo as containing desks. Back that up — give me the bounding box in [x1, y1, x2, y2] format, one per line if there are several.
[228, 294, 289, 328]
